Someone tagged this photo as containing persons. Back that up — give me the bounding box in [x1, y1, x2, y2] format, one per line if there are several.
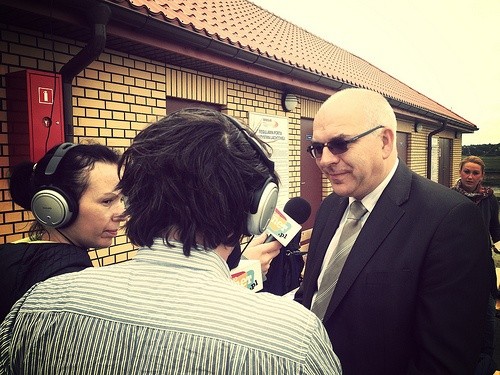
[293, 88, 500, 375]
[0, 106, 343, 375]
[0, 137, 281, 325]
[448, 155, 500, 253]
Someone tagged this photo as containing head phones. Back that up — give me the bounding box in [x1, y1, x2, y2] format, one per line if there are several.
[32, 142, 79, 229]
[178, 107, 279, 236]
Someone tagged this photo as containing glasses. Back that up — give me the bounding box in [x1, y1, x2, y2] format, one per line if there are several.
[306, 124, 385, 160]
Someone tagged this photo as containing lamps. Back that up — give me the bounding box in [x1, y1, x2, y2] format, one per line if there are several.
[282, 92, 298, 112]
[415, 122, 423, 133]
[455, 130, 461, 139]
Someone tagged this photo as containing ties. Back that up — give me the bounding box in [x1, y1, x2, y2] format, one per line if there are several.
[311, 201, 369, 323]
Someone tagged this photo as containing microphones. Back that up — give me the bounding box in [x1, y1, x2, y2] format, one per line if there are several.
[262, 197, 311, 247]
[225, 240, 264, 292]
